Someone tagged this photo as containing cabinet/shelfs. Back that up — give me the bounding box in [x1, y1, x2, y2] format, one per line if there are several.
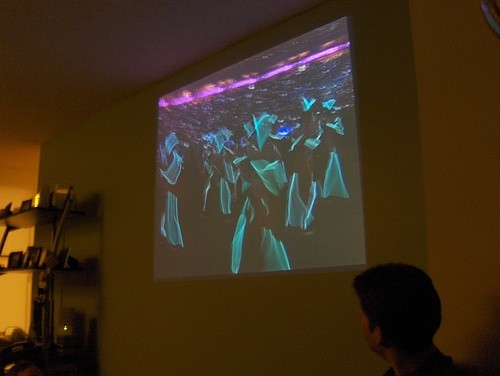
[0, 206, 100, 376]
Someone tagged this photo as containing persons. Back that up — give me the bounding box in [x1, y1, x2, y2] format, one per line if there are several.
[354, 262, 477, 375]
